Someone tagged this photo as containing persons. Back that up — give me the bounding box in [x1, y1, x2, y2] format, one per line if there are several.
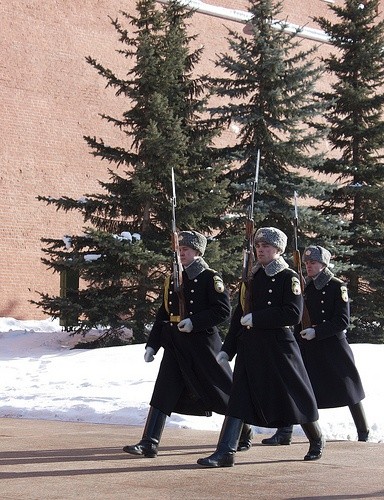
[197, 227, 326, 468]
[122, 230, 255, 458]
[260, 246, 370, 445]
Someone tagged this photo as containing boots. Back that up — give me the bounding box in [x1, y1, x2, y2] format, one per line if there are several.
[261, 425, 294, 445]
[298, 421, 325, 461]
[237, 423, 253, 451]
[349, 402, 369, 443]
[123, 407, 167, 458]
[197, 416, 244, 466]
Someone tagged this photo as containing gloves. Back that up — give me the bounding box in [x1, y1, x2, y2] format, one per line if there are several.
[216, 351, 229, 366]
[300, 328, 316, 340]
[144, 347, 156, 362]
[241, 313, 253, 328]
[177, 318, 193, 333]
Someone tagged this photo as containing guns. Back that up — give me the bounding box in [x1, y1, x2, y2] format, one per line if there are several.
[171, 167, 186, 322]
[244, 149, 261, 319]
[292, 191, 313, 329]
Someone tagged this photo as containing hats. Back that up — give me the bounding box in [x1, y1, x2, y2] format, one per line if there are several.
[255, 227, 288, 255]
[302, 246, 332, 266]
[178, 231, 208, 257]
